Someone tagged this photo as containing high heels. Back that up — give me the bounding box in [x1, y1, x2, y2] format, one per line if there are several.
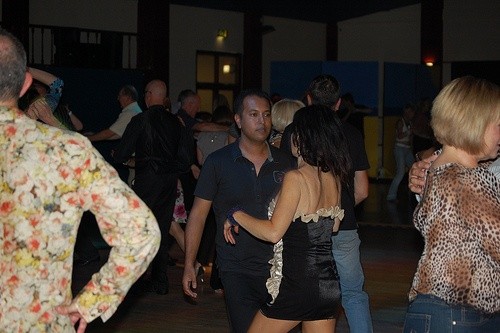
[196, 266, 205, 283]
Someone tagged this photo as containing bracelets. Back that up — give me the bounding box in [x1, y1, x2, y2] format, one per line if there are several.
[227, 206, 246, 224]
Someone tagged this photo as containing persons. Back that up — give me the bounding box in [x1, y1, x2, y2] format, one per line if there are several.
[18, 66, 418, 301]
[409, 132, 499, 200]
[402, 78, 500, 332]
[280, 77, 375, 332]
[267, 97, 306, 149]
[182, 88, 296, 333]
[0, 31, 162, 332]
[223, 104, 351, 332]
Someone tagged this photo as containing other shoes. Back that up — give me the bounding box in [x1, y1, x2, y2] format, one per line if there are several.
[214, 289, 224, 296]
[157, 286, 169, 295]
[74, 253, 100, 266]
